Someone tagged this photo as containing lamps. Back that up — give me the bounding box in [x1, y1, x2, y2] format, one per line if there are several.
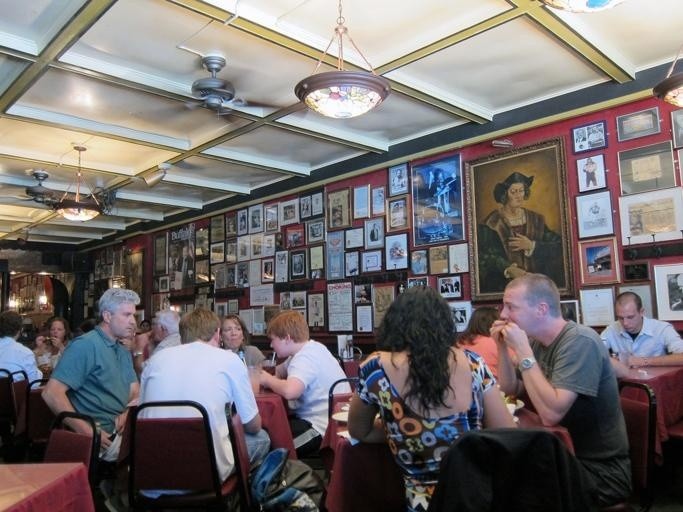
[44, 144, 103, 223]
[533, 0, 629, 17]
[292, 0, 392, 123]
[651, 44, 683, 109]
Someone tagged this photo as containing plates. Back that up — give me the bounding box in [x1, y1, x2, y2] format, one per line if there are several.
[514, 398, 524, 412]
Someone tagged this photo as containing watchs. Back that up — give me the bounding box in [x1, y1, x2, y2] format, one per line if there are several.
[519, 356, 537, 373]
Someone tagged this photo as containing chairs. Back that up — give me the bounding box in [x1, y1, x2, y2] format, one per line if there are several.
[0, 342, 682, 512]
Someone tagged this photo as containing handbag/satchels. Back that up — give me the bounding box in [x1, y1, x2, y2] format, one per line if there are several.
[252, 449, 326, 512]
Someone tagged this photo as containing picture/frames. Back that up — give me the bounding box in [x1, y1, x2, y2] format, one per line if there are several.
[371, 282, 396, 329]
[679, 148, 682, 185]
[620, 260, 652, 283]
[447, 300, 472, 333]
[326, 228, 344, 282]
[409, 248, 429, 277]
[360, 249, 384, 273]
[617, 140, 678, 196]
[560, 300, 581, 325]
[579, 285, 616, 329]
[578, 236, 621, 286]
[344, 250, 362, 280]
[77, 189, 308, 340]
[429, 243, 449, 276]
[616, 107, 662, 141]
[305, 219, 326, 245]
[671, 107, 682, 150]
[371, 185, 387, 217]
[575, 154, 607, 193]
[310, 186, 328, 221]
[353, 304, 375, 335]
[449, 241, 469, 274]
[384, 193, 411, 232]
[325, 187, 351, 232]
[619, 186, 682, 246]
[408, 150, 467, 251]
[616, 281, 656, 318]
[325, 279, 356, 333]
[463, 135, 577, 303]
[407, 276, 428, 289]
[306, 291, 327, 332]
[436, 274, 464, 300]
[308, 243, 324, 278]
[300, 192, 313, 222]
[571, 120, 609, 154]
[384, 232, 411, 272]
[387, 162, 410, 197]
[363, 217, 385, 251]
[574, 188, 613, 240]
[352, 272, 408, 302]
[344, 224, 364, 252]
[353, 184, 371, 220]
[653, 264, 683, 321]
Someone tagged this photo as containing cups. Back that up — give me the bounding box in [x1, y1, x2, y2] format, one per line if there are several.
[505, 403, 513, 415]
[248, 367, 259, 394]
[260, 359, 276, 392]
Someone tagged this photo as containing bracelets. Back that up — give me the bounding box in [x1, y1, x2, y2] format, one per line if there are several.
[642, 357, 648, 367]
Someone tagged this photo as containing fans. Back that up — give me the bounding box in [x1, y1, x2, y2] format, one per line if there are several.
[129, 53, 308, 130]
[0, 171, 61, 204]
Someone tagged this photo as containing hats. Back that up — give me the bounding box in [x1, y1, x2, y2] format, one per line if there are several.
[500, 172, 533, 189]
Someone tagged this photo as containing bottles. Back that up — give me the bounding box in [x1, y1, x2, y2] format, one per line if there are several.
[237, 350, 245, 362]
[346, 335, 354, 358]
[341, 348, 347, 358]
[611, 352, 619, 361]
[134, 350, 143, 381]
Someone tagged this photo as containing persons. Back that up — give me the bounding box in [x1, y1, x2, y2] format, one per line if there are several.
[489, 273, 634, 507]
[370, 223, 378, 241]
[294, 256, 303, 272]
[459, 305, 518, 379]
[431, 168, 457, 204]
[264, 263, 273, 278]
[589, 127, 603, 147]
[582, 157, 597, 187]
[347, 283, 518, 511]
[478, 172, 565, 294]
[600, 291, 683, 381]
[393, 169, 404, 186]
[178, 245, 193, 282]
[576, 129, 586, 143]
